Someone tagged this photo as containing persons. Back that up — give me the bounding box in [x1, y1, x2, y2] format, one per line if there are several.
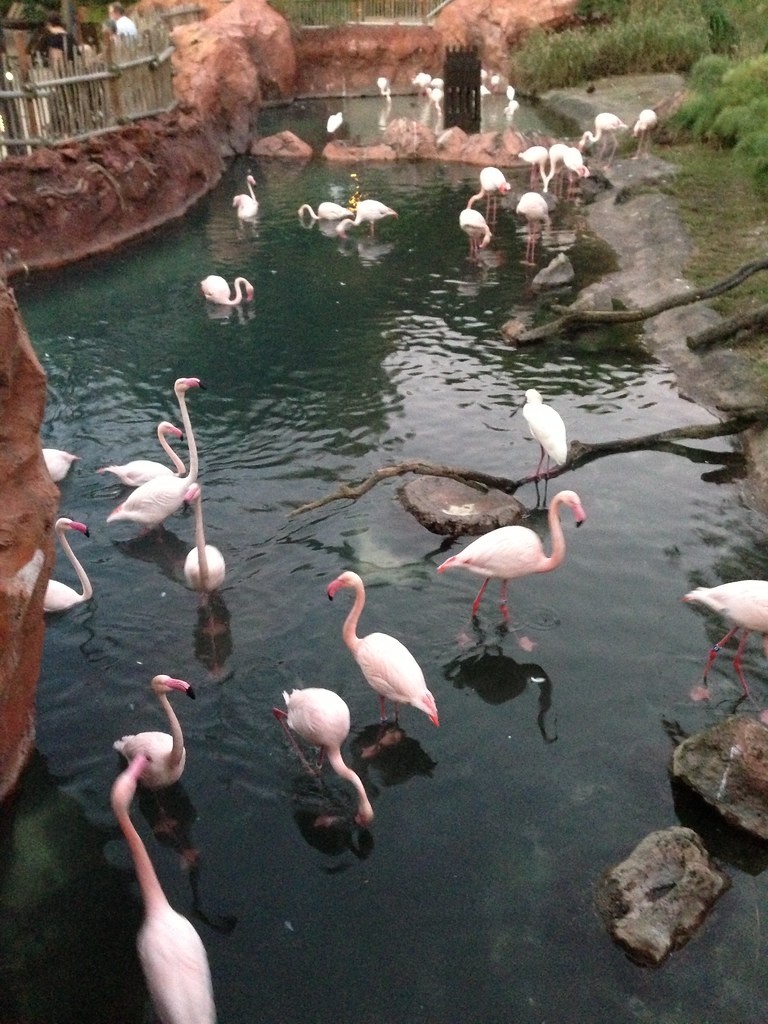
[34, 2, 138, 134]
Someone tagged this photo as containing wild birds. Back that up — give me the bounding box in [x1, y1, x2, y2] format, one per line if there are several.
[521, 389, 567, 479]
[233, 175, 260, 219]
[515, 192, 551, 237]
[273, 688, 374, 828]
[326, 69, 659, 193]
[299, 202, 353, 221]
[437, 489, 585, 623]
[682, 579, 767, 700]
[328, 570, 440, 728]
[106, 378, 206, 532]
[112, 673, 197, 790]
[335, 200, 400, 238]
[111, 754, 217, 1024]
[466, 167, 510, 213]
[200, 274, 255, 306]
[42, 449, 81, 482]
[184, 482, 225, 597]
[42, 518, 94, 613]
[459, 209, 492, 249]
[94, 422, 187, 486]
